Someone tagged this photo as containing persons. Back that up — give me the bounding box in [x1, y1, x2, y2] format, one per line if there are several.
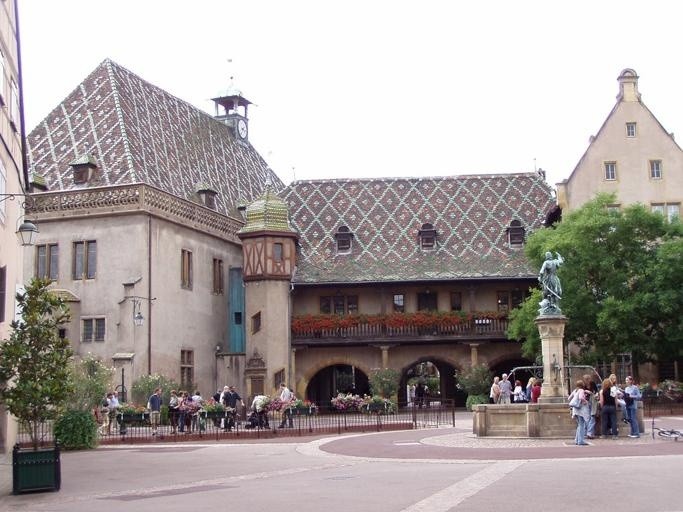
[95, 380, 297, 432]
[488, 371, 643, 446]
[537, 250, 564, 308]
[424, 388, 430, 408]
[414, 382, 424, 407]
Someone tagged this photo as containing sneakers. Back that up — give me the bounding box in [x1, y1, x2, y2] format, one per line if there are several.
[576, 435, 640, 446]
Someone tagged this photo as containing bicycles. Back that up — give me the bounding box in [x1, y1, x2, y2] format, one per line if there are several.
[650, 414, 682, 444]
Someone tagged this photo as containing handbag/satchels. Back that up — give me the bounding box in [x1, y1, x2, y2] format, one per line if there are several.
[569, 389, 585, 409]
